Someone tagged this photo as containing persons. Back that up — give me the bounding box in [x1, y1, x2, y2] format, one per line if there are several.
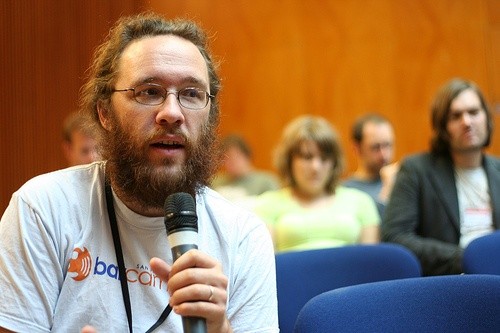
[0, 16, 279, 333]
[380, 80, 500, 276]
[215, 135, 278, 196]
[61, 111, 102, 165]
[339, 115, 400, 223]
[260, 115, 381, 252]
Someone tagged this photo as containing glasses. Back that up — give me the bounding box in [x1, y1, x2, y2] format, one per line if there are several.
[112, 82, 215, 109]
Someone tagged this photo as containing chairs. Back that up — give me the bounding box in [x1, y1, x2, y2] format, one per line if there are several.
[275, 230, 500, 333]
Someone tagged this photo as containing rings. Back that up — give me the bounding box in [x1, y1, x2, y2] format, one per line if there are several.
[208, 284, 215, 301]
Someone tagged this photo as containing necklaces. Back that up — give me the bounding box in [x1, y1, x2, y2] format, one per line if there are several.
[105, 159, 197, 333]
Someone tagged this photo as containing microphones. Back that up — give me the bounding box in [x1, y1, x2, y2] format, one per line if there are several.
[164, 192, 207, 333]
